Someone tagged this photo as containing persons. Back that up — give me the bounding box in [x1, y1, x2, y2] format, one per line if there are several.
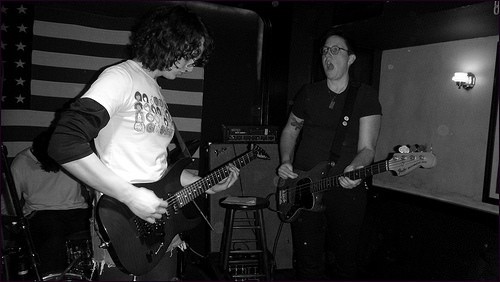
[1, 133, 91, 262]
[46, 9, 213, 282]
[278, 29, 382, 280]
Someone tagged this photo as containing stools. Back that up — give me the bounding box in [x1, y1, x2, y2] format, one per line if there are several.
[219, 196, 271, 282]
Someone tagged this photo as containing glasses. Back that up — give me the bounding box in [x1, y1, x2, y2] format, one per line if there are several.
[187, 58, 197, 67]
[320, 45, 349, 55]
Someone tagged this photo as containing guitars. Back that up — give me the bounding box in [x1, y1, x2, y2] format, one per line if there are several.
[275, 145, 437, 224]
[95, 141, 272, 275]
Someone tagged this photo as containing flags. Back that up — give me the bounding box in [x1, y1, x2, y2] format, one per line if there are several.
[0, 0, 204, 159]
